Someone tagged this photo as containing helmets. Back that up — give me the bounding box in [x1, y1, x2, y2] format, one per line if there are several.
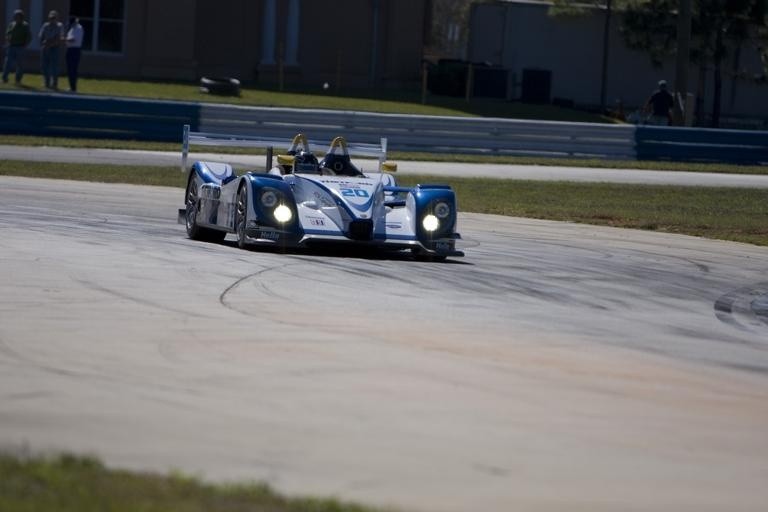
[294, 152, 320, 173]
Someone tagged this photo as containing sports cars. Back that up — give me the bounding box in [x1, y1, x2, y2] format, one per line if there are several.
[174, 133, 466, 265]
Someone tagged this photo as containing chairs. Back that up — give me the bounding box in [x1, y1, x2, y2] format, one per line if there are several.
[282, 134, 352, 176]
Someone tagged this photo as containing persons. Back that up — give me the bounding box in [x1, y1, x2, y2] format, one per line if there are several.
[0, 10, 34, 85]
[37, 9, 63, 88]
[639, 79, 675, 123]
[59, 15, 86, 93]
